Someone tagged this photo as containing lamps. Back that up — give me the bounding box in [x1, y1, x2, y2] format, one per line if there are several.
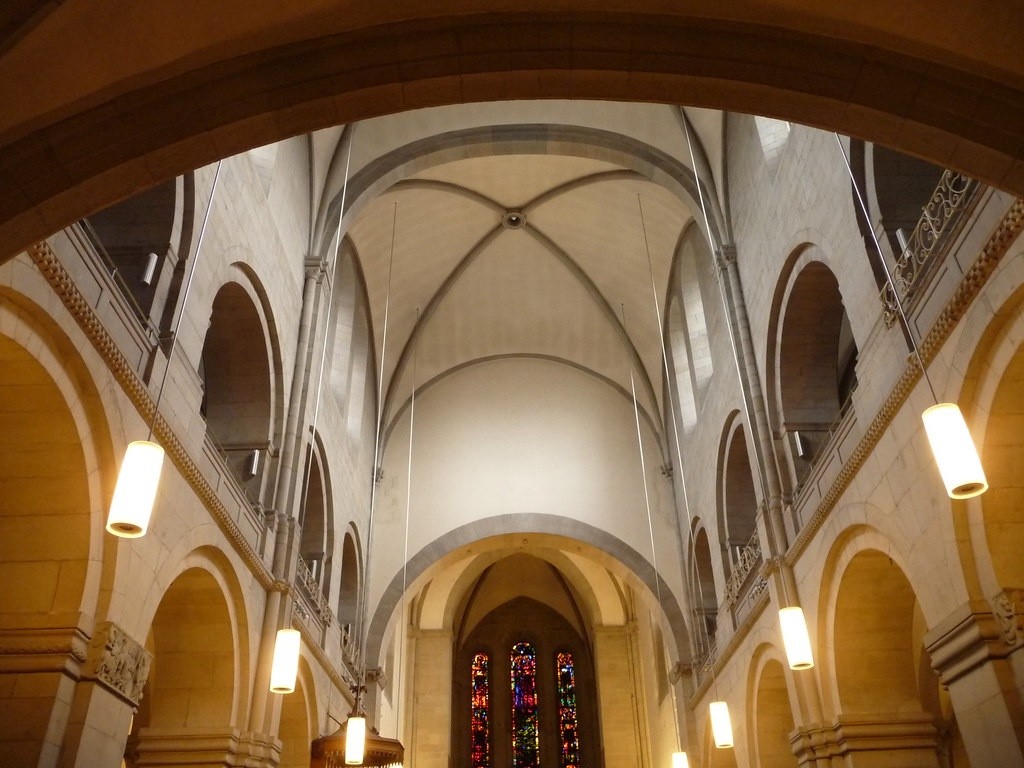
[621, 302, 690, 768]
[637, 193, 735, 749]
[676, 106, 814, 671]
[269, 121, 356, 695]
[106, 160, 223, 539]
[834, 133, 989, 500]
[345, 202, 398, 765]
